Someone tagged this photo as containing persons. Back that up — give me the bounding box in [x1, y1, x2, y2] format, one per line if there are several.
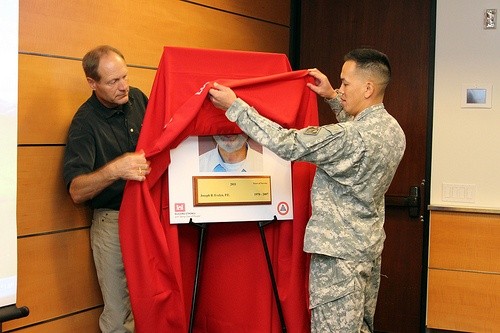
[198, 134, 263, 172]
[207, 49, 406, 333]
[62, 45, 153, 333]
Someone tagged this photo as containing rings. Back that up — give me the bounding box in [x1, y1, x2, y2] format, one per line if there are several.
[139, 167, 142, 175]
